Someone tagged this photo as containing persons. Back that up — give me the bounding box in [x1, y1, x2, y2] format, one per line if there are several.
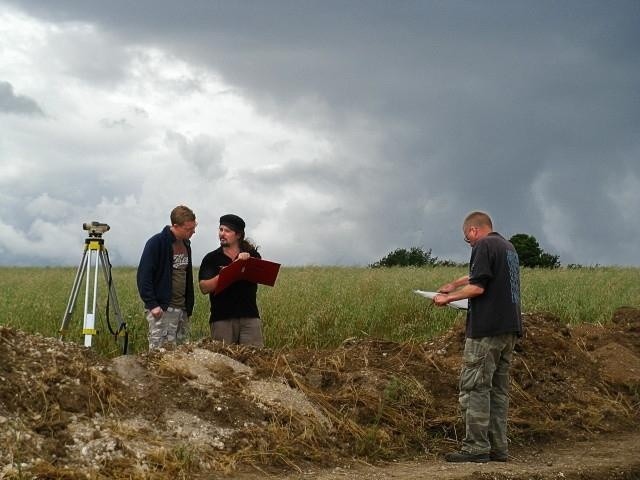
[433, 211, 522, 463]
[199, 215, 262, 347]
[136, 205, 197, 350]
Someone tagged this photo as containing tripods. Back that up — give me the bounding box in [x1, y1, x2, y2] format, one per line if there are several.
[58, 237, 129, 355]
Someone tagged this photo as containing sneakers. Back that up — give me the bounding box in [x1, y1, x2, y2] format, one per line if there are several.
[489, 448, 509, 463]
[445, 450, 490, 464]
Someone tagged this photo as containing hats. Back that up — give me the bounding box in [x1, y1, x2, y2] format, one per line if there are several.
[220, 214, 246, 232]
